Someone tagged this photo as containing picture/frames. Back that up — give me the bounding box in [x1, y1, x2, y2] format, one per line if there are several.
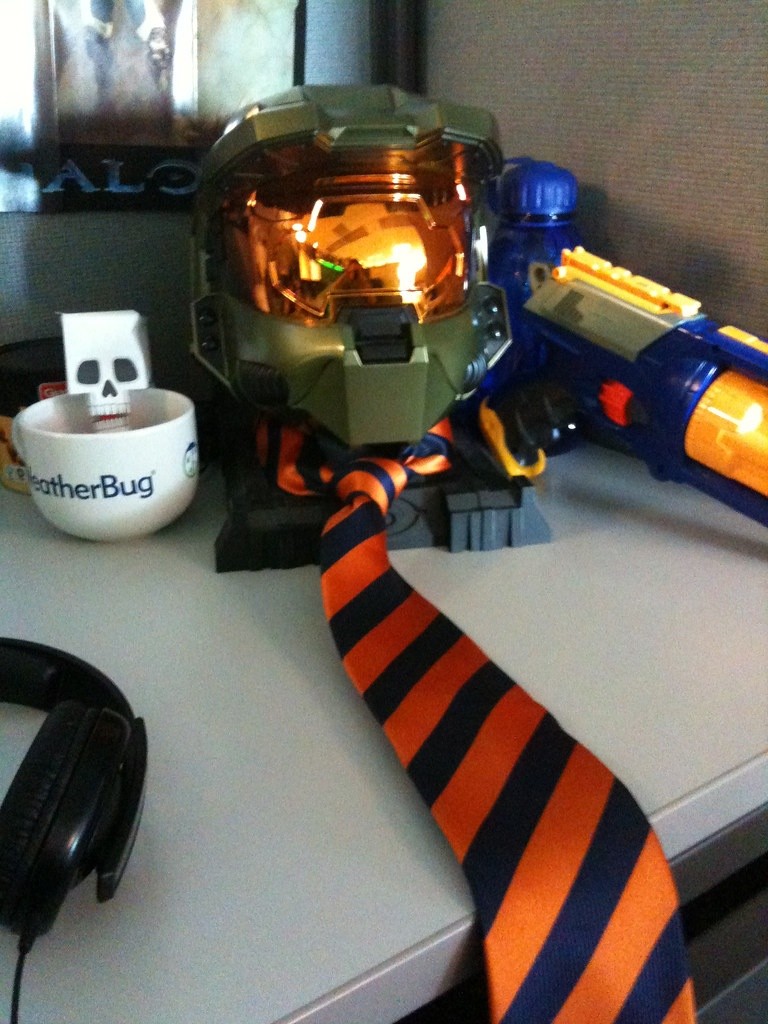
[33, 0, 198, 190]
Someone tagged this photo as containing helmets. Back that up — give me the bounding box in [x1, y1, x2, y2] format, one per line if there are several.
[190, 81, 511, 445]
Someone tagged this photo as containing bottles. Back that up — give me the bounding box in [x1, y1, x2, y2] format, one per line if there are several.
[487, 156, 580, 366]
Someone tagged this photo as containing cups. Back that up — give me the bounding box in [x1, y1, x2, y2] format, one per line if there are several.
[11, 388, 199, 542]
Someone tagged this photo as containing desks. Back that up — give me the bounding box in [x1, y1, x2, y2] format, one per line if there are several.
[0, 441, 768, 1024]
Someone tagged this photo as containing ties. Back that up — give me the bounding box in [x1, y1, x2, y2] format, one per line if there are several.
[254, 416, 696, 1024]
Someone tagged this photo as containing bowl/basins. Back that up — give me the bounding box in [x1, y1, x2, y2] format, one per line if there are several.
[0, 336, 67, 495]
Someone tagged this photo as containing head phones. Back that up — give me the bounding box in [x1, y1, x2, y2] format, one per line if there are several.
[0, 637, 150, 948]
